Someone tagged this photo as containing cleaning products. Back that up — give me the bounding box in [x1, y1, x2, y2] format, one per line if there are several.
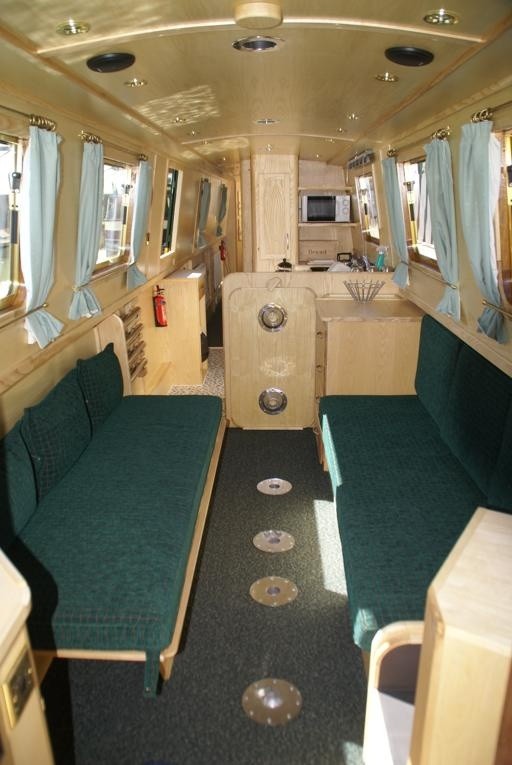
[376, 247, 387, 272]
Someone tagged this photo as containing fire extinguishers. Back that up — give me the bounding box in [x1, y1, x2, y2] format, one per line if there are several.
[154, 285, 167, 327]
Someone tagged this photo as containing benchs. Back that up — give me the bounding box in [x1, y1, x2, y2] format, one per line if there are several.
[319, 314, 512, 682]
[0, 322, 229, 697]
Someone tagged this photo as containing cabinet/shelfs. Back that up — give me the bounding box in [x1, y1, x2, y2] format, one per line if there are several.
[162, 269, 208, 385]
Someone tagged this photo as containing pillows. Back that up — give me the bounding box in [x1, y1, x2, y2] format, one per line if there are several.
[77, 343, 124, 430]
[20, 368, 93, 503]
[0, 420, 38, 553]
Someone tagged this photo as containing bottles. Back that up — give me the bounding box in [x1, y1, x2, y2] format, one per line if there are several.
[375, 251, 385, 272]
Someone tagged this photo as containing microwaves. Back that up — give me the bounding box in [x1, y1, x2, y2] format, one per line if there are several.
[301, 195, 350, 223]
